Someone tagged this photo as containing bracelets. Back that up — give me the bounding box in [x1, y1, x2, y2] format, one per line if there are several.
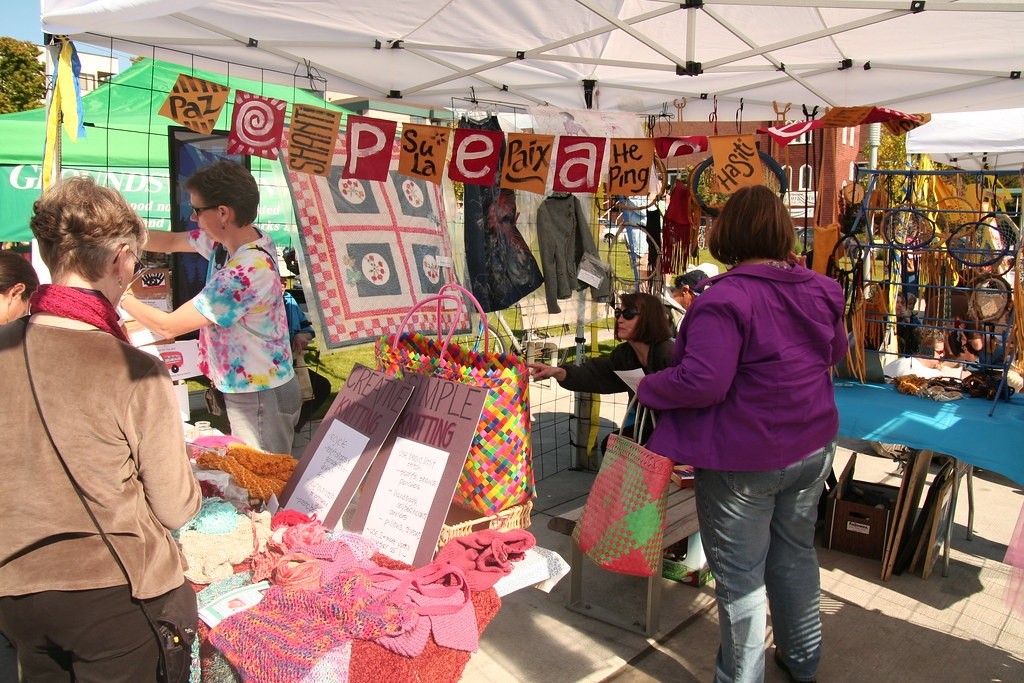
[119, 293, 131, 308]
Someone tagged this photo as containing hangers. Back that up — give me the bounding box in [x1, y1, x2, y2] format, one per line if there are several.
[552, 191, 567, 197]
[462, 101, 492, 122]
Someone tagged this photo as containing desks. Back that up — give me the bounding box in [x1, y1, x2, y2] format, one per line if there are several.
[835, 380, 1024, 488]
[183, 423, 570, 683]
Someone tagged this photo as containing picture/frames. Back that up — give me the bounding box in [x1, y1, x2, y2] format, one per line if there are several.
[880, 448, 961, 581]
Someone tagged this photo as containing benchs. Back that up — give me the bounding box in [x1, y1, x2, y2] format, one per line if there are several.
[517, 290, 629, 387]
[547, 481, 702, 637]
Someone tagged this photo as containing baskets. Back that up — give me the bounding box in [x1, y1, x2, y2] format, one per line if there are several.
[435, 501, 533, 548]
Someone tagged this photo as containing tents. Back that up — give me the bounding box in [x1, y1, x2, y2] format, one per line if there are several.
[40, 1, 1024, 191]
[905, 109, 1024, 171]
[0, 58, 360, 167]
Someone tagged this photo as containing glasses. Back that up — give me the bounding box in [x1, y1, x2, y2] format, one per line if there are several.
[614, 307, 642, 320]
[188, 203, 227, 217]
[113, 243, 146, 279]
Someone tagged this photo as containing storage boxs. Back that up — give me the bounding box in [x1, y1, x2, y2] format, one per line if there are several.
[846, 327, 896, 380]
[824, 453, 899, 559]
[663, 530, 715, 588]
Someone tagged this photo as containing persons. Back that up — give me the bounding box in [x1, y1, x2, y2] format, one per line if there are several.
[0, 248, 39, 326]
[527, 293, 677, 457]
[0, 176, 203, 682]
[636, 185, 848, 683]
[661, 270, 710, 331]
[896, 292, 1003, 364]
[283, 292, 331, 447]
[118, 159, 303, 457]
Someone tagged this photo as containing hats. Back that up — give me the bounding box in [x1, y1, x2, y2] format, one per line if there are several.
[686, 263, 718, 278]
[677, 270, 712, 296]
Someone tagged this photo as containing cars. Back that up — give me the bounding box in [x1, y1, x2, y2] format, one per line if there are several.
[795, 226, 862, 259]
[602, 226, 627, 245]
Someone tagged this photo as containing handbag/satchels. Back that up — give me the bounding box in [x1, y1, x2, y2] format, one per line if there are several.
[572, 391, 676, 577]
[151, 575, 199, 683]
[374, 284, 538, 518]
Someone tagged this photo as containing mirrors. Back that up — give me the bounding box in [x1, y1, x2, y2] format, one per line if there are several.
[168, 125, 251, 340]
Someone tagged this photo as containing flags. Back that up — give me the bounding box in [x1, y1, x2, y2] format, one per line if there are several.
[449, 127, 505, 186]
[399, 122, 450, 186]
[227, 90, 287, 161]
[502, 133, 555, 195]
[288, 103, 342, 177]
[553, 136, 606, 193]
[342, 114, 397, 182]
[655, 134, 708, 158]
[708, 133, 764, 194]
[757, 105, 931, 148]
[158, 74, 230, 135]
[608, 138, 655, 197]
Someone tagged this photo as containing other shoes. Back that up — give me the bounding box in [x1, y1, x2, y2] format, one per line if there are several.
[774, 646, 817, 683]
